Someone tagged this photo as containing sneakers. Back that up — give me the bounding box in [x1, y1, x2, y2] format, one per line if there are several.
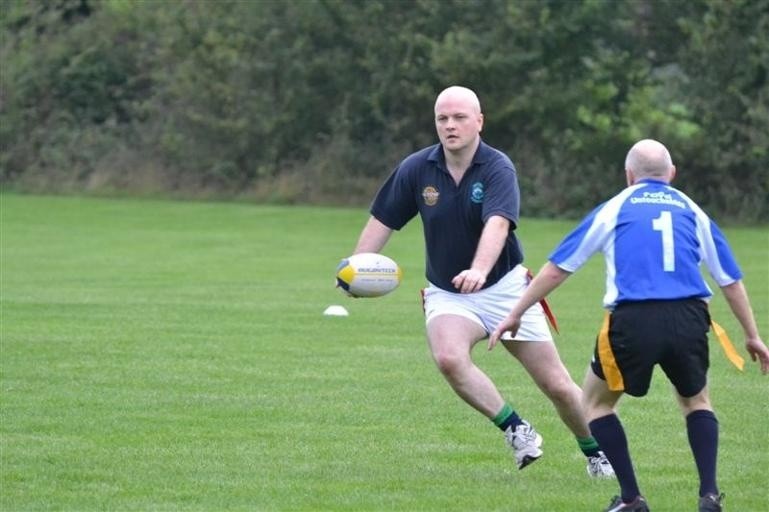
[603, 495, 647, 511]
[698, 493, 723, 512]
[505, 419, 543, 469]
[586, 451, 617, 479]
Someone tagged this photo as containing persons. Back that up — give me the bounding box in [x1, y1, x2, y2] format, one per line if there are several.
[336, 84, 616, 480]
[484, 136, 769, 511]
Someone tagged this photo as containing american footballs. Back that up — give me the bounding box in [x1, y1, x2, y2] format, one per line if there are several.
[336, 252, 402, 297]
